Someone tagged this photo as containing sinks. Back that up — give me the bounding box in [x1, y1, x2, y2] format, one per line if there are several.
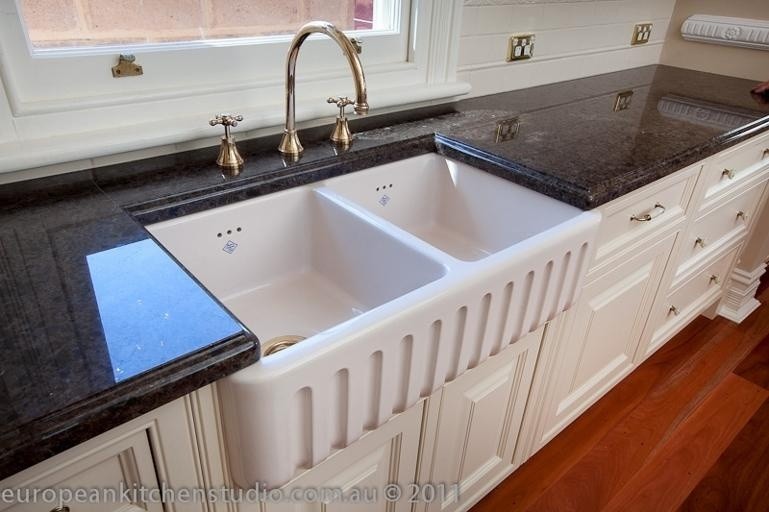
[143, 190, 449, 385]
[322, 150, 586, 263]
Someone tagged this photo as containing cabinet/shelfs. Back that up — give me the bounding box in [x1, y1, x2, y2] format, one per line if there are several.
[528, 128, 769, 461]
[0, 380, 235, 512]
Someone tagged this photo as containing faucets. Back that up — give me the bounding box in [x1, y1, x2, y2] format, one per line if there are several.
[278, 22, 369, 154]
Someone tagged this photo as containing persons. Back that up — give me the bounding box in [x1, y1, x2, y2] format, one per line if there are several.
[750, 93, 769, 110]
[750, 80, 769, 94]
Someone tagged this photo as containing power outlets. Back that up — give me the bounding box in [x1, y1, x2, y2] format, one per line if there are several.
[613, 91, 633, 112]
[507, 33, 535, 62]
[495, 117, 520, 144]
[631, 24, 653, 45]
